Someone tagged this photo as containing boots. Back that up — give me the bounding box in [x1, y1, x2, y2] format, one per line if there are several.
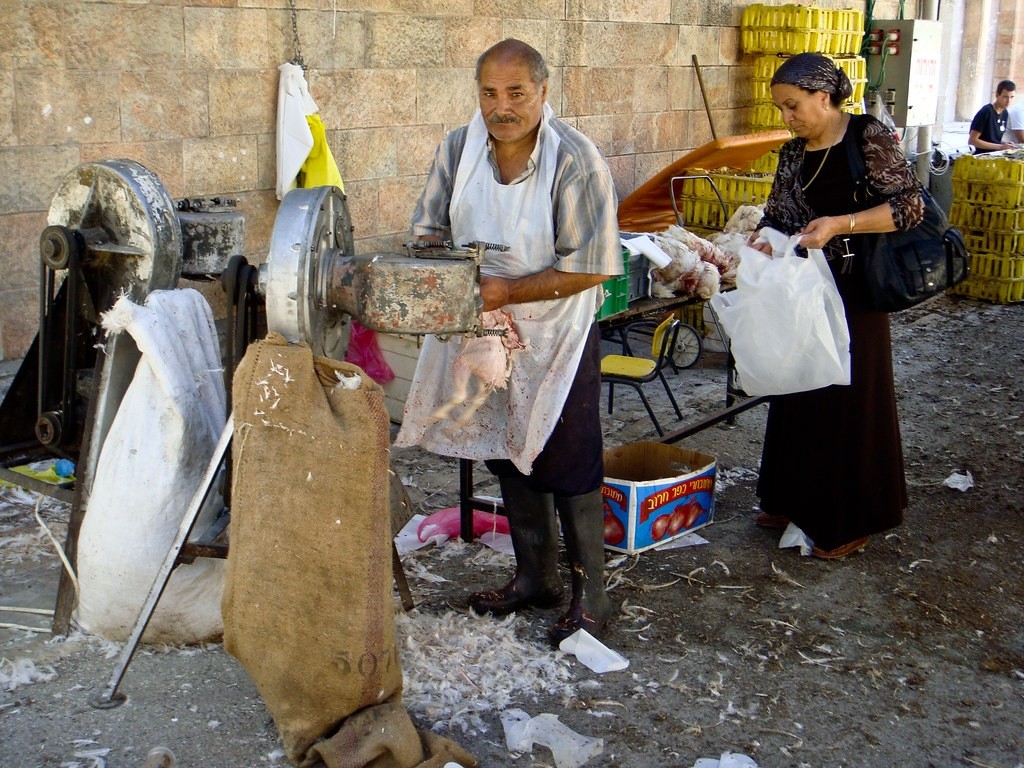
[547, 486, 609, 646]
[469, 491, 565, 616]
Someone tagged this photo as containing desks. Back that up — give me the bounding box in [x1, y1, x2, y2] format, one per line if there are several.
[598, 282, 734, 441]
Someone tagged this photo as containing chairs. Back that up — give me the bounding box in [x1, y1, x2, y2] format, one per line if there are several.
[601, 313, 683, 438]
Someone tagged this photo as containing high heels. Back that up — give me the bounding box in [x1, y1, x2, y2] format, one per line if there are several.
[811, 537, 869, 559]
[756, 512, 789, 526]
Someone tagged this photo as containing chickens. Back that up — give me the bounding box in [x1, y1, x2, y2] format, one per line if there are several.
[425, 309, 526, 432]
[648, 204, 766, 300]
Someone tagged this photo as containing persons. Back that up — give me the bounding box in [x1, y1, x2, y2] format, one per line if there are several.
[747, 52, 928, 560]
[393, 37, 626, 647]
[968, 80, 1024, 155]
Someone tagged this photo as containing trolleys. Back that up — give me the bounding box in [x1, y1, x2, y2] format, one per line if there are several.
[666, 174, 750, 401]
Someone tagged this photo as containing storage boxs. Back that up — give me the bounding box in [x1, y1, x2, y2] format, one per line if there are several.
[678, 3, 868, 340]
[946, 157, 1024, 305]
[601, 440, 717, 556]
[595, 230, 661, 322]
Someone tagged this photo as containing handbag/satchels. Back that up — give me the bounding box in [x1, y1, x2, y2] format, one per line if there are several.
[848, 113, 970, 312]
